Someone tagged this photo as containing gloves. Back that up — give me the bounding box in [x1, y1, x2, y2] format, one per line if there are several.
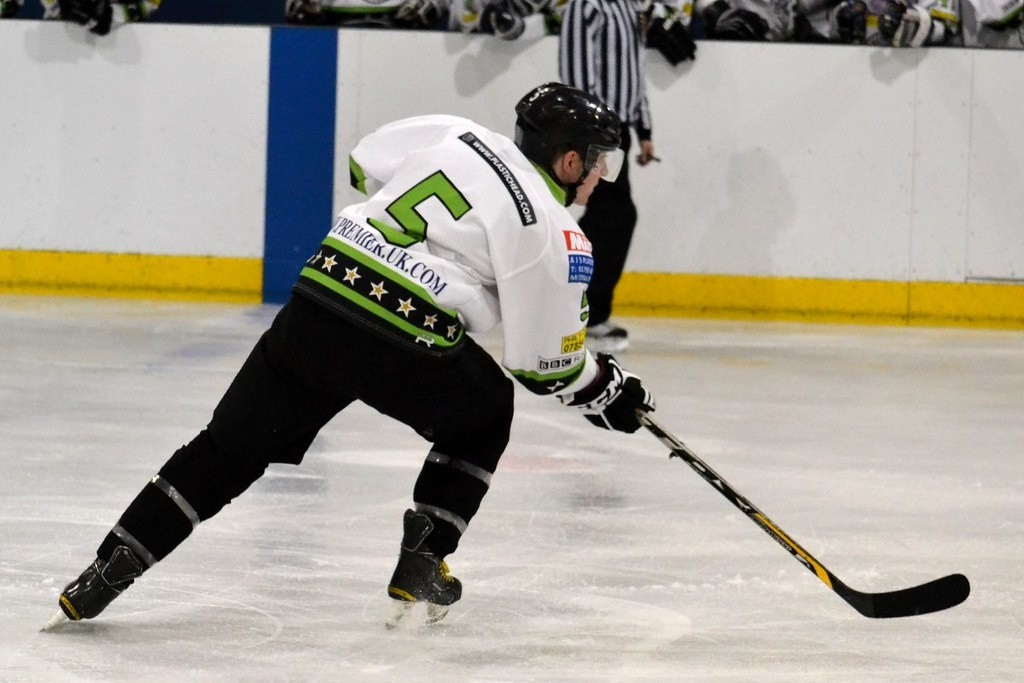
[557, 352, 655, 433]
[645, 18, 697, 66]
[878, 2, 936, 48]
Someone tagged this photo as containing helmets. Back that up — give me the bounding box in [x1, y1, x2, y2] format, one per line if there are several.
[515, 82, 621, 195]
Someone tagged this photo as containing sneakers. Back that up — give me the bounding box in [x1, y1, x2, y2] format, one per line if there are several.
[43, 545, 143, 631]
[585, 322, 630, 354]
[385, 509, 462, 631]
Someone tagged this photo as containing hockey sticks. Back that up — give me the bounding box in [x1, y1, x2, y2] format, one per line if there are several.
[635, 407, 974, 621]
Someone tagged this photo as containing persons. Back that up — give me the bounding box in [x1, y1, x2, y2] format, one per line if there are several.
[0, 0, 1024, 65]
[38, 80, 656, 632]
[560, 0, 654, 355]
